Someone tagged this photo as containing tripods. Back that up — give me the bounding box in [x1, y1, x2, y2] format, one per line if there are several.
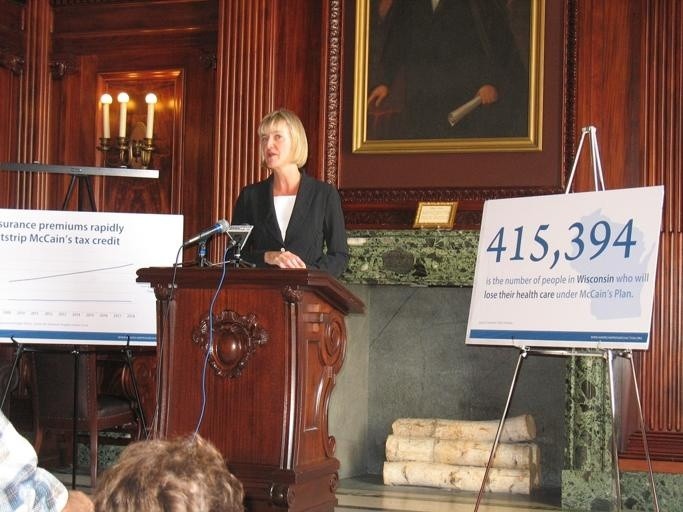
[172, 242, 213, 268]
[208, 242, 256, 269]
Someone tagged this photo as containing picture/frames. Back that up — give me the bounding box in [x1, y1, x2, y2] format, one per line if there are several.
[352, 1, 544, 155]
[413, 202, 459, 229]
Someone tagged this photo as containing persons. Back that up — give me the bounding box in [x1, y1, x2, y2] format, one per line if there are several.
[0, 406, 94, 512]
[229, 107, 350, 280]
[89, 430, 247, 512]
[367, 0, 502, 138]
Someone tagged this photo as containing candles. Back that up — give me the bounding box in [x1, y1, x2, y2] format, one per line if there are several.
[100, 92, 158, 139]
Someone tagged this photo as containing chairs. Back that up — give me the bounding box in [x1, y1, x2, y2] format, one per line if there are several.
[30, 347, 141, 493]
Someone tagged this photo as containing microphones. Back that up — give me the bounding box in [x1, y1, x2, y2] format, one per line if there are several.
[226, 234, 242, 249]
[182, 219, 229, 246]
